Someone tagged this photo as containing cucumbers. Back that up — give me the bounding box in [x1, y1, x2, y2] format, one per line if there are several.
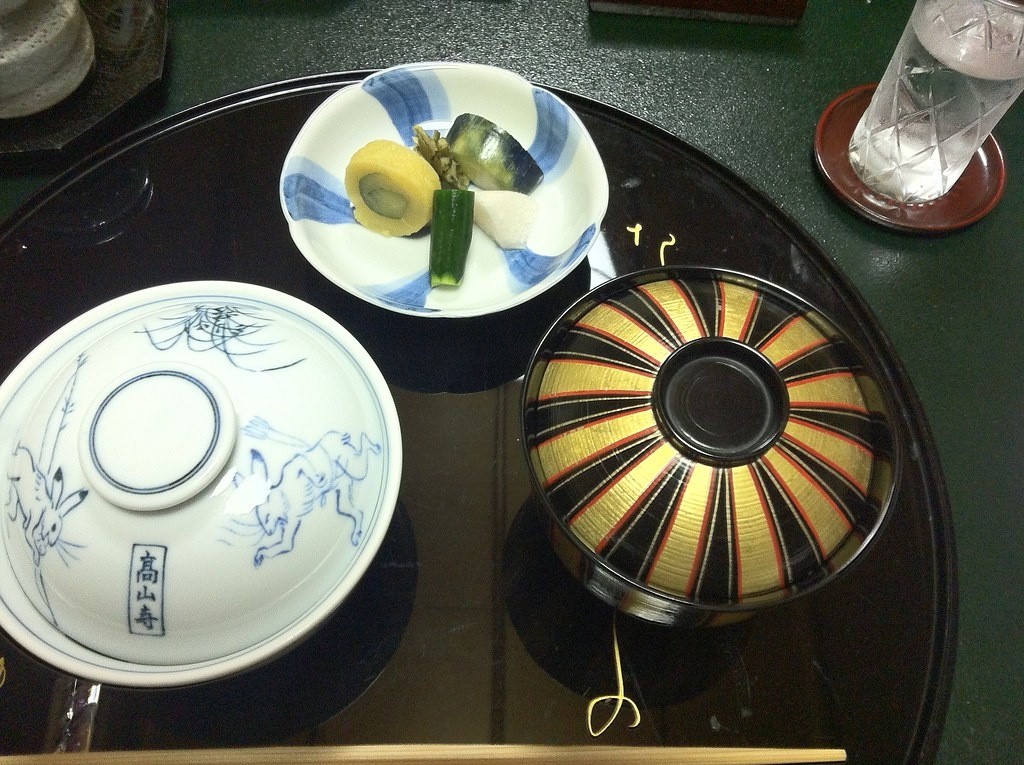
[428, 189, 474, 286]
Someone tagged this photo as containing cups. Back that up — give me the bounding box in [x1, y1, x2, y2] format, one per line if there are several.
[0, 1, 94, 118]
[848, 1, 1024, 207]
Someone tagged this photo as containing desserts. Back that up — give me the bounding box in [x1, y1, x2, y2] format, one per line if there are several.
[446, 113, 552, 249]
[345, 139, 441, 237]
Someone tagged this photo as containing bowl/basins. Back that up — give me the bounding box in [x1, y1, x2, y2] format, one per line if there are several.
[0, 281, 403, 691]
[520, 265, 903, 629]
[280, 61, 609, 318]
